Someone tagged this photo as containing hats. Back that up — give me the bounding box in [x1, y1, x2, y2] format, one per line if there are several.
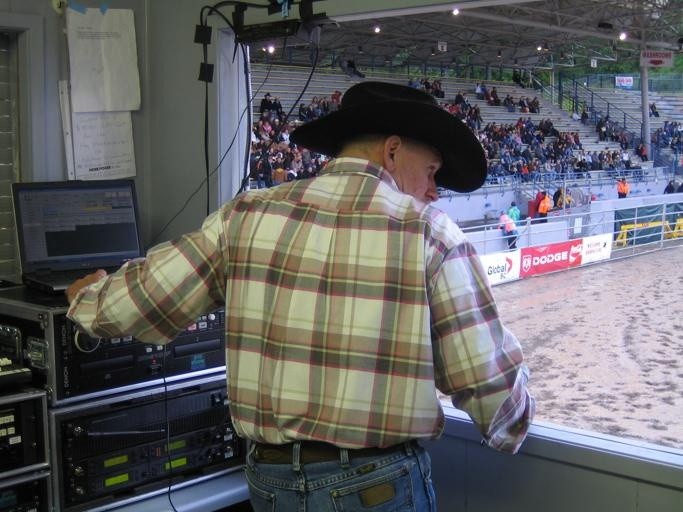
[283, 79, 495, 196]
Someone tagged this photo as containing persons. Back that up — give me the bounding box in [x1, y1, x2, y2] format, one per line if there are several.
[553, 187, 572, 208]
[408, 76, 444, 98]
[439, 89, 482, 129]
[664, 179, 683, 194]
[474, 82, 541, 113]
[509, 202, 521, 224]
[654, 120, 683, 154]
[60, 86, 532, 512]
[250, 91, 342, 188]
[618, 178, 629, 198]
[513, 73, 540, 89]
[473, 117, 650, 183]
[533, 189, 551, 224]
[649, 102, 658, 116]
[491, 209, 518, 248]
[572, 100, 588, 122]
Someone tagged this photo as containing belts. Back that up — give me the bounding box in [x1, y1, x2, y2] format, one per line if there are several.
[249, 436, 419, 466]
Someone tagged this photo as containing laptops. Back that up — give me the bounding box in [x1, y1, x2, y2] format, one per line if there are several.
[10, 178, 146, 295]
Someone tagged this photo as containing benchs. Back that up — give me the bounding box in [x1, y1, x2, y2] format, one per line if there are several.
[250, 63, 682, 154]
